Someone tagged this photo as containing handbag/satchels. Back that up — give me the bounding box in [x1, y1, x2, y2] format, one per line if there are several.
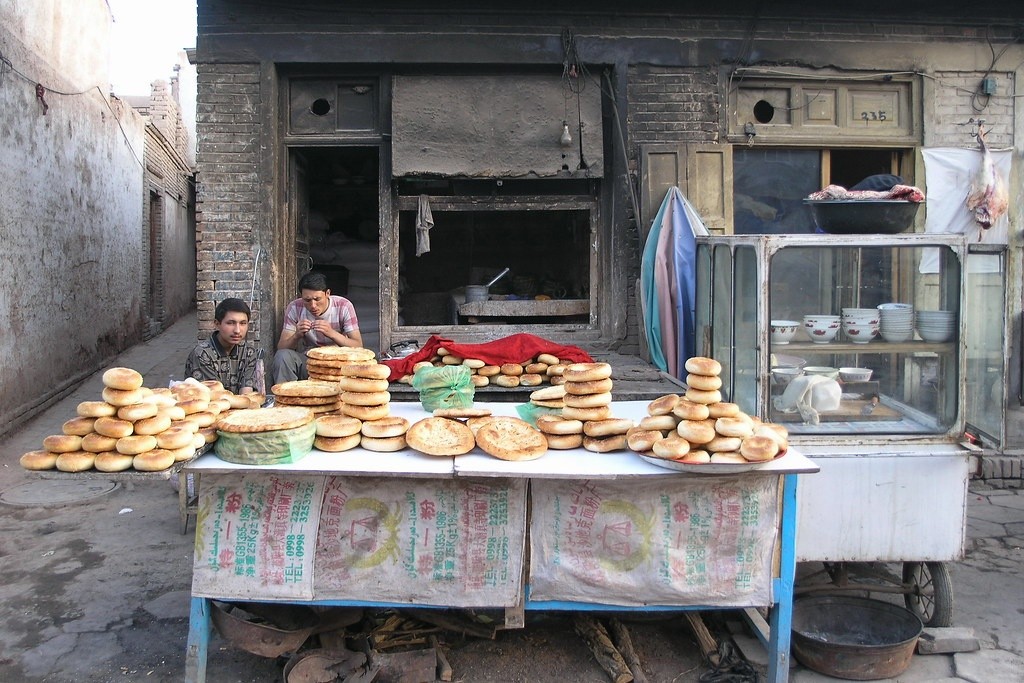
[215, 419, 316, 465]
[515, 400, 563, 431]
[412, 366, 476, 413]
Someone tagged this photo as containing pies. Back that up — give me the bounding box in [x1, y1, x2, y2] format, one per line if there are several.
[215, 380, 341, 464]
[626, 357, 790, 463]
[405, 407, 549, 461]
[20, 367, 266, 471]
[529, 362, 633, 452]
[315, 364, 410, 452]
[305, 347, 376, 382]
[399, 346, 574, 387]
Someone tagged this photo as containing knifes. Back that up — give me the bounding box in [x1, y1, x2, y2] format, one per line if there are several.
[862, 397, 877, 413]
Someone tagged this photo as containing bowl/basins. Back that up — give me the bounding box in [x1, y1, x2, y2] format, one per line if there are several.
[282, 647, 366, 683]
[837, 367, 873, 383]
[770, 354, 807, 370]
[786, 594, 924, 680]
[802, 198, 927, 233]
[803, 366, 840, 381]
[772, 368, 805, 387]
[768, 303, 959, 345]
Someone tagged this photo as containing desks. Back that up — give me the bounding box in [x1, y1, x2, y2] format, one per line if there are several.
[29, 396, 824, 683]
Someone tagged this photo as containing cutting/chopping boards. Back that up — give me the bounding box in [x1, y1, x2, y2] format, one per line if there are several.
[771, 396, 903, 420]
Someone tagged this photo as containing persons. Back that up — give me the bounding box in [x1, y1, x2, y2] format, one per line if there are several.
[271, 273, 362, 385]
[183, 297, 258, 394]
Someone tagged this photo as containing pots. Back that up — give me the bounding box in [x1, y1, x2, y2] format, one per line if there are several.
[209, 599, 365, 657]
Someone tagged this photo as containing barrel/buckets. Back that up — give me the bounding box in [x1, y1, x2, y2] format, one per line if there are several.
[465, 284, 488, 305]
